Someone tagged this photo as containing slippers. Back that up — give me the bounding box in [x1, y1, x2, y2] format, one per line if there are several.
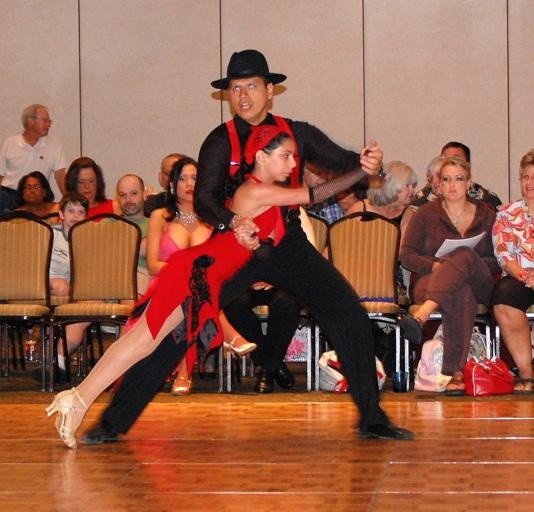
[512, 378, 533, 394]
[401, 314, 423, 345]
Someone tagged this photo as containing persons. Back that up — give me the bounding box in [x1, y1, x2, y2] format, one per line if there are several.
[40, 123, 386, 454]
[77, 46, 417, 444]
[1, 102, 68, 215]
[300, 142, 534, 398]
[17, 153, 258, 399]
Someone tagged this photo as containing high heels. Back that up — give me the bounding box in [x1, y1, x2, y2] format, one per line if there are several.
[45, 386, 89, 448]
[171, 375, 193, 395]
[223, 333, 257, 359]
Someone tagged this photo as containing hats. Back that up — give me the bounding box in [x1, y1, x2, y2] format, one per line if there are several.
[211, 49, 286, 90]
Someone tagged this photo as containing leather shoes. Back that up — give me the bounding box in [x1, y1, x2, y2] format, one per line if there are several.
[58, 366, 70, 383]
[80, 424, 119, 443]
[256, 366, 274, 392]
[274, 362, 294, 389]
[360, 423, 412, 440]
[200, 371, 215, 380]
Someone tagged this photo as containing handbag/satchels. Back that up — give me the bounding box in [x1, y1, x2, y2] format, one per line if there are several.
[319, 348, 386, 395]
[414, 324, 516, 396]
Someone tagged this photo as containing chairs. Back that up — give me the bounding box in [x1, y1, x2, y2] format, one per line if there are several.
[214, 211, 403, 395]
[0, 213, 141, 391]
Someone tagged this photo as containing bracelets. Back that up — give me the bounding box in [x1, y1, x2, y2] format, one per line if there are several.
[231, 215, 242, 226]
